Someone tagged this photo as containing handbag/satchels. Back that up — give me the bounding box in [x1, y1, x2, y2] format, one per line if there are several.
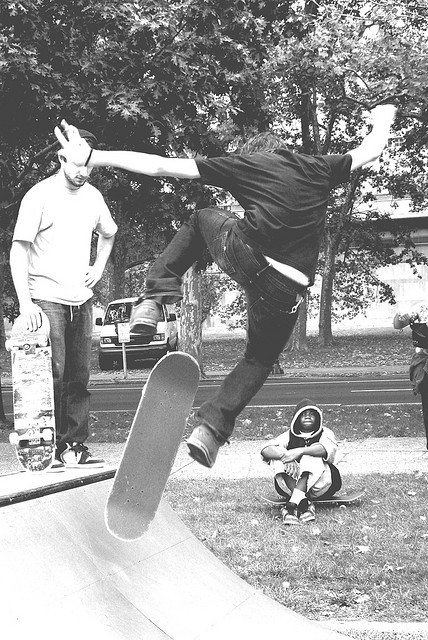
[409, 321, 428, 348]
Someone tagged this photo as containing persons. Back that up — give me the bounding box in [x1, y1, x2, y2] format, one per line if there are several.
[9, 129, 118, 474]
[392, 303, 428, 452]
[52, 101, 397, 468]
[261, 399, 341, 525]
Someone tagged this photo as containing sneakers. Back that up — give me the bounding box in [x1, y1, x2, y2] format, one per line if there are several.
[187, 424, 224, 469]
[46, 444, 65, 473]
[281, 502, 298, 525]
[62, 442, 105, 468]
[298, 498, 315, 522]
[129, 299, 160, 337]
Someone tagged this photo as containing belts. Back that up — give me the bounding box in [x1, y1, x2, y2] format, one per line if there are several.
[246, 242, 306, 294]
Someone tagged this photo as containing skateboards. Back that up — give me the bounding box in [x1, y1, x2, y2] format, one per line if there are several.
[260, 491, 365, 510]
[104, 351, 200, 541]
[5, 311, 56, 473]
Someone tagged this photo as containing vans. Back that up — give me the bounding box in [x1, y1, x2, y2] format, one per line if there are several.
[95, 297, 179, 371]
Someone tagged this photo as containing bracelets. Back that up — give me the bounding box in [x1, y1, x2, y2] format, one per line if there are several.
[83, 148, 95, 168]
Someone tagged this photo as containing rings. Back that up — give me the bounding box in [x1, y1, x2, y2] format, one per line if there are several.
[296, 466, 300, 470]
